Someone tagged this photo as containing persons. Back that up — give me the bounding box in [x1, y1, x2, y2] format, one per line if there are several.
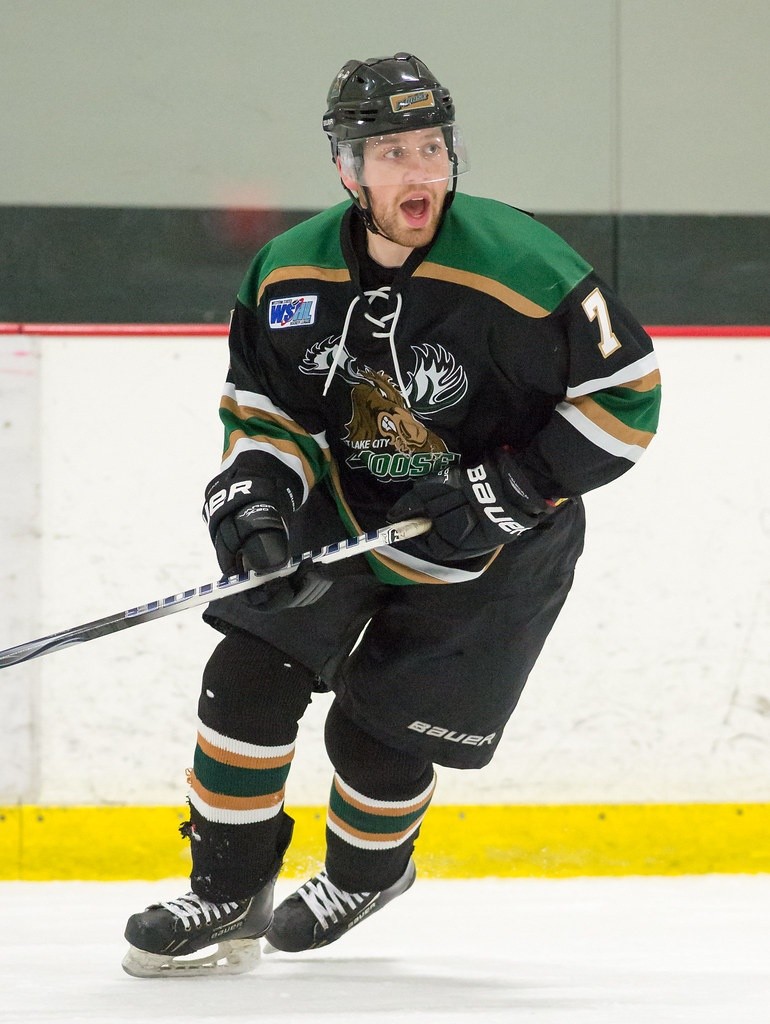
[122, 53, 662, 979]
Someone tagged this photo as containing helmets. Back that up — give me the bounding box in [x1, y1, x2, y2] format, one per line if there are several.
[321, 51, 471, 185]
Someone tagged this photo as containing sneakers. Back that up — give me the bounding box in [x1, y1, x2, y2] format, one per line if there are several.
[124, 887, 272, 977]
[265, 867, 418, 959]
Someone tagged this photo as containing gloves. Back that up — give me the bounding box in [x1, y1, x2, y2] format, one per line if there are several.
[203, 479, 301, 606]
[388, 451, 555, 563]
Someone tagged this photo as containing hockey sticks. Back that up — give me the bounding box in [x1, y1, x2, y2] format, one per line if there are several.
[1, 518, 432, 671]
